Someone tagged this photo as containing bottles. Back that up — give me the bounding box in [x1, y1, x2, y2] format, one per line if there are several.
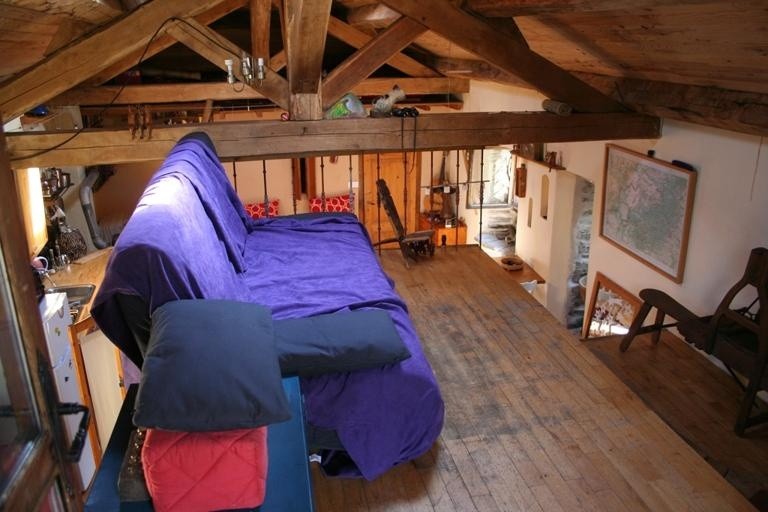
[48, 245, 64, 271]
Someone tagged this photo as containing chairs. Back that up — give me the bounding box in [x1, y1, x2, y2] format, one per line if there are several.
[621, 247, 767, 439]
[372, 177, 436, 268]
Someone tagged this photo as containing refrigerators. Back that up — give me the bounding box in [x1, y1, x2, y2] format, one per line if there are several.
[38, 291, 103, 489]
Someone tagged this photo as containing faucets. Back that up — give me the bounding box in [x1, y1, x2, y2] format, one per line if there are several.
[37, 269, 58, 289]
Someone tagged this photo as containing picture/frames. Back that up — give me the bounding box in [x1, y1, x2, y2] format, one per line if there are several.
[581, 144, 697, 340]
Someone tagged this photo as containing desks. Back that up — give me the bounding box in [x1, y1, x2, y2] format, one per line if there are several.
[87, 375, 313, 511]
[40, 247, 113, 324]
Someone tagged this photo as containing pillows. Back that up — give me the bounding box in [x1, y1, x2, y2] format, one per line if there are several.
[275, 310, 411, 377]
[136, 301, 294, 428]
[242, 191, 356, 220]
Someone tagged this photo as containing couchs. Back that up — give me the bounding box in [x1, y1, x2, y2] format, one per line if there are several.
[93, 130, 444, 485]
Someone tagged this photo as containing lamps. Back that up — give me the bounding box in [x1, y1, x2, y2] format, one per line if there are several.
[222, 51, 267, 93]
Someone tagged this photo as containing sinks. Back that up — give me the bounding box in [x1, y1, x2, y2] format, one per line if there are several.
[69, 306, 83, 325]
[45, 284, 96, 303]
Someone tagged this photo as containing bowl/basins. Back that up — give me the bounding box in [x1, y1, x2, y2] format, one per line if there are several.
[501, 257, 524, 271]
